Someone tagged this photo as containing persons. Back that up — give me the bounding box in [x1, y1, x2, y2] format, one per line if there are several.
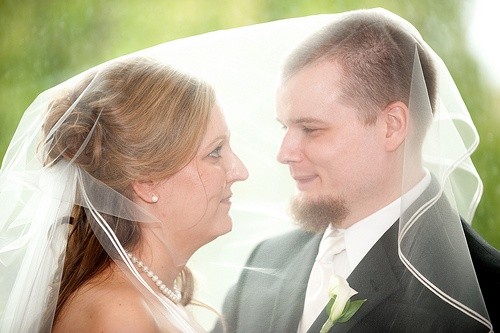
[208, 12, 500, 333]
[21, 57, 250, 333]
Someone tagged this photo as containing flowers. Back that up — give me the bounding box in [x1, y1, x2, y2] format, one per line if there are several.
[319, 248, 368, 333]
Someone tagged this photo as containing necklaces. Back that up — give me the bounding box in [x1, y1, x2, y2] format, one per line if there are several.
[124, 250, 182, 304]
